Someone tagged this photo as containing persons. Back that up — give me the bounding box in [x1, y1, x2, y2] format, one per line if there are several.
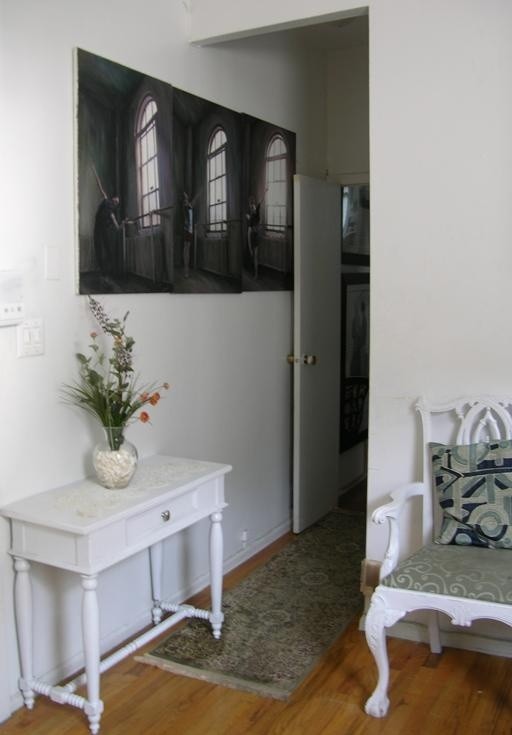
[90, 163, 124, 287]
[246, 186, 268, 282]
[181, 189, 201, 278]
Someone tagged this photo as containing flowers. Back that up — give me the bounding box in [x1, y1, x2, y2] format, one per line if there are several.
[58, 295, 169, 450]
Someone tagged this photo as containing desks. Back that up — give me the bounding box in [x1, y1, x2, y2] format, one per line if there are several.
[0, 454, 233, 735]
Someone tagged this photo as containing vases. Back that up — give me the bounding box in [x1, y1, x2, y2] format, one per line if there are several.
[91, 426, 139, 490]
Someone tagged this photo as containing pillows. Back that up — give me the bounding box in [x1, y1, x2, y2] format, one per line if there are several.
[428, 441, 512, 548]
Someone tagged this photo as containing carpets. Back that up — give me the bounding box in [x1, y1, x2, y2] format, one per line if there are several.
[133, 509, 365, 700]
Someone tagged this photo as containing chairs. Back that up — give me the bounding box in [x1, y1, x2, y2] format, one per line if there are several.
[365, 393, 512, 716]
